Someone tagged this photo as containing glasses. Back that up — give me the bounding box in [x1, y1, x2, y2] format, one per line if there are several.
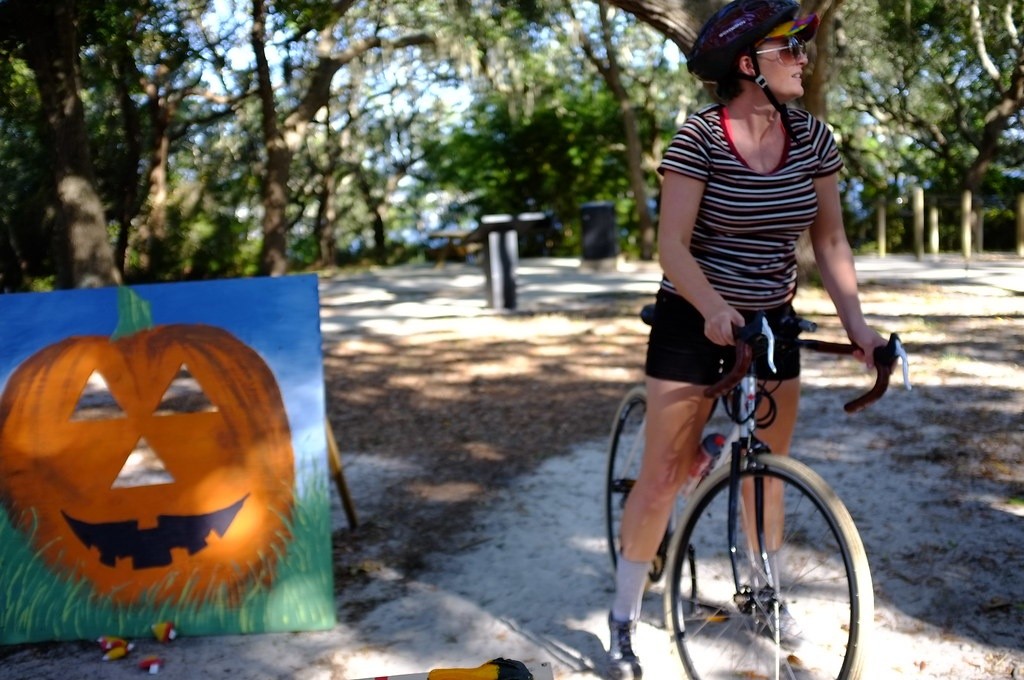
[752, 35, 808, 63]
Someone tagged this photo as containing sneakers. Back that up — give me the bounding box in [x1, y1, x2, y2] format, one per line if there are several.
[608, 607, 643, 680]
[758, 599, 802, 649]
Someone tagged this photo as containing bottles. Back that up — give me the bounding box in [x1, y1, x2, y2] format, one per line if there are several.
[688, 433, 725, 479]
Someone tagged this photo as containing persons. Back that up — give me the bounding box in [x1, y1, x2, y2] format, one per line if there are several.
[606, 0, 897, 680]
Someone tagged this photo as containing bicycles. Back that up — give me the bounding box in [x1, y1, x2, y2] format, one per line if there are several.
[603, 304, 914, 680]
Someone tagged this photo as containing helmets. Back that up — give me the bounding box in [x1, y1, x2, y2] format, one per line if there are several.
[686, 0, 799, 83]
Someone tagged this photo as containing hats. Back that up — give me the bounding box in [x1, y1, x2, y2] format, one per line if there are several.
[754, 14, 821, 48]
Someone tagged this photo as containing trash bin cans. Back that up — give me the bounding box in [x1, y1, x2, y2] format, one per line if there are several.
[579, 201, 619, 270]
